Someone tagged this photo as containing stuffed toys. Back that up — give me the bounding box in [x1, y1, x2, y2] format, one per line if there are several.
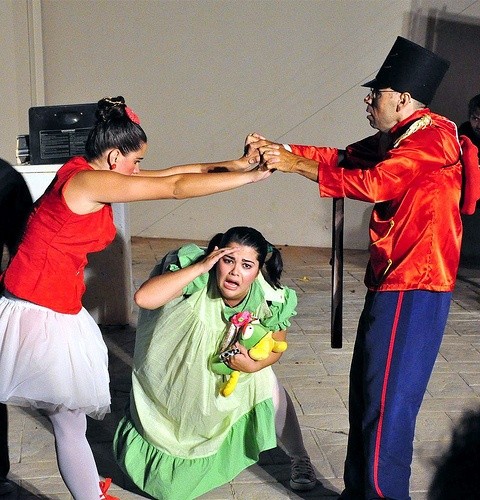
[208, 318, 287, 398]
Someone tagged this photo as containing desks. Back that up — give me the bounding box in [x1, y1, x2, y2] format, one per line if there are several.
[14, 163, 130, 328]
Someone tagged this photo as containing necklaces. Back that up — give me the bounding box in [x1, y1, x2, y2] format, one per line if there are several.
[224, 298, 233, 307]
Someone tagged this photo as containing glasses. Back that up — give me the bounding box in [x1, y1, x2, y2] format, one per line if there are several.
[370, 88, 395, 99]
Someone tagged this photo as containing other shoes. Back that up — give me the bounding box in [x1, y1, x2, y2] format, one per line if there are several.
[290, 456, 316, 489]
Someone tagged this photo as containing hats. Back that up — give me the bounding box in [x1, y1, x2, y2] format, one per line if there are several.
[361, 36, 450, 106]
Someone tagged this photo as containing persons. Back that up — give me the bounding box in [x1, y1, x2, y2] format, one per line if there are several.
[1, 156, 34, 500]
[113, 226, 318, 500]
[459, 95, 480, 157]
[0, 97, 281, 500]
[245, 36, 465, 499]
